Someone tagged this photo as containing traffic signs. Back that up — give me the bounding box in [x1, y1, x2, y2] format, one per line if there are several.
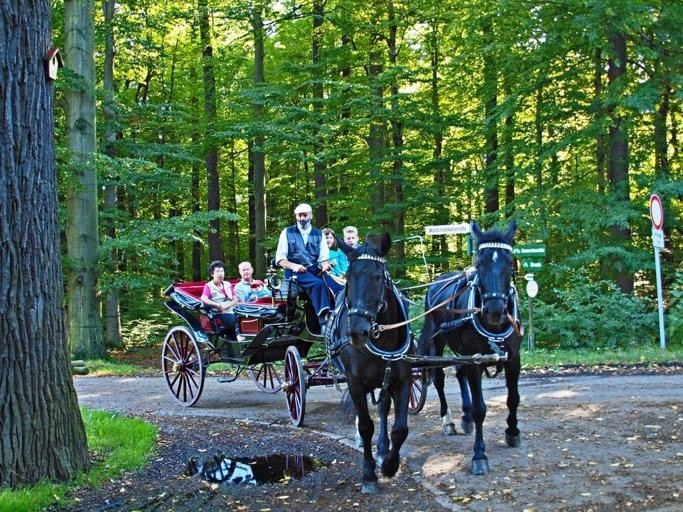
[510, 244, 547, 258]
[424, 221, 471, 236]
[520, 258, 543, 274]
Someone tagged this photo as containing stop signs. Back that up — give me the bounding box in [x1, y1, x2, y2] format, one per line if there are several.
[649, 194, 664, 231]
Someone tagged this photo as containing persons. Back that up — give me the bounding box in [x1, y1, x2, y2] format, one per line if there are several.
[274, 201, 347, 336]
[232, 261, 272, 305]
[199, 259, 248, 343]
[341, 226, 361, 250]
[318, 226, 350, 281]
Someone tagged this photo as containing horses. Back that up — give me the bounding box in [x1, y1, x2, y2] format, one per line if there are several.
[415, 218, 522, 475]
[323, 231, 416, 496]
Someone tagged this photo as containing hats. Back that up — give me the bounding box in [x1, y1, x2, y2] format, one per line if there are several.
[294, 203, 312, 214]
[250, 280, 264, 286]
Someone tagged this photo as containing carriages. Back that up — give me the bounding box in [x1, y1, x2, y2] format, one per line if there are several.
[161, 219, 526, 498]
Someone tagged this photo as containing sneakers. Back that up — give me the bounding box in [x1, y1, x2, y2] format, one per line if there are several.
[237, 334, 245, 343]
